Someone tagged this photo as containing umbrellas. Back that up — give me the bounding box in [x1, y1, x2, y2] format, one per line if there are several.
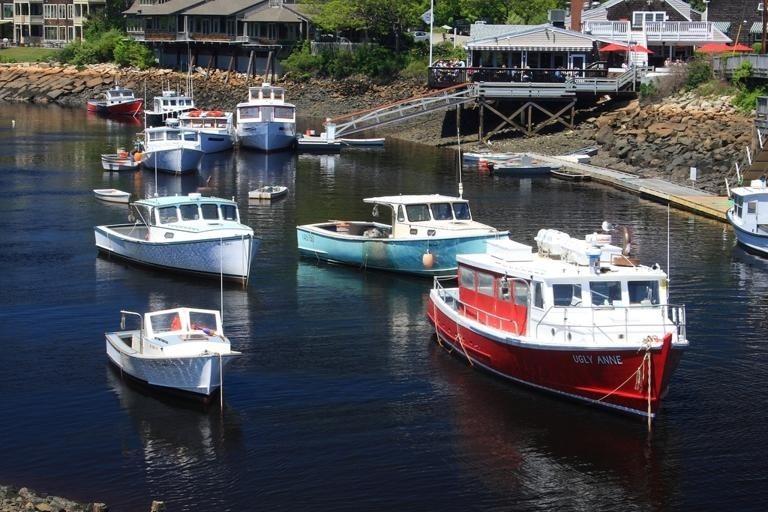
[598, 44, 632, 51]
[696, 44, 730, 53]
[729, 43, 753, 50]
[631, 45, 653, 53]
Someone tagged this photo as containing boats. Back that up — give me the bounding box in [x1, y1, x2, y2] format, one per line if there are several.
[87, 49, 387, 177]
[92, 187, 134, 205]
[720, 173, 768, 261]
[102, 234, 244, 414]
[422, 198, 693, 427]
[247, 181, 289, 202]
[457, 144, 595, 185]
[92, 129, 257, 289]
[294, 100, 513, 281]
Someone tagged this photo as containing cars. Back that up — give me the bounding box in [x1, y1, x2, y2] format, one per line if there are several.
[447, 18, 472, 35]
[411, 31, 429, 45]
[315, 34, 351, 43]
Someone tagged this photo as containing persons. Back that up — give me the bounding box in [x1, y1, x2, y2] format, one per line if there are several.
[432, 58, 581, 83]
[663, 58, 687, 67]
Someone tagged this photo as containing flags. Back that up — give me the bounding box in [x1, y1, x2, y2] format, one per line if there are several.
[421, 10, 434, 25]
